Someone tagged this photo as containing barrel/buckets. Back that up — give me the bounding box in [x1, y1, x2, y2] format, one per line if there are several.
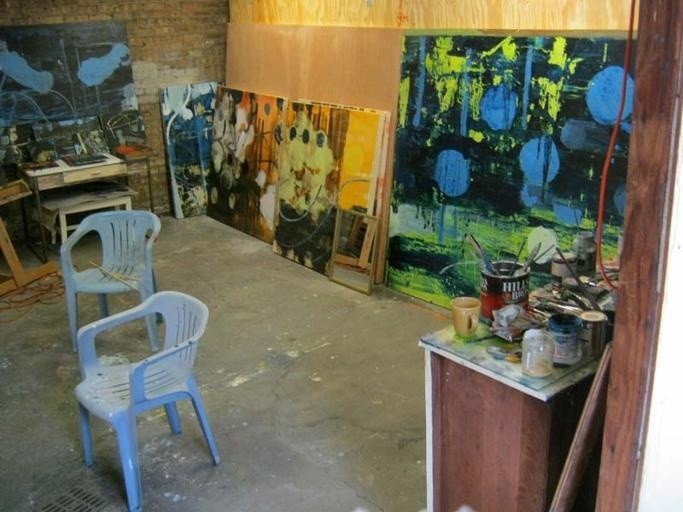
[551, 254, 574, 277]
[574, 229, 598, 276]
[481, 261, 531, 324]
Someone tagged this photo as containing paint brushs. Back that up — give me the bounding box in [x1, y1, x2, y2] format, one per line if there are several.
[466, 234, 555, 276]
[524, 293, 582, 328]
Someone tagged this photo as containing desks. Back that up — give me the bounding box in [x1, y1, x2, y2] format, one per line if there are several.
[17, 150, 155, 265]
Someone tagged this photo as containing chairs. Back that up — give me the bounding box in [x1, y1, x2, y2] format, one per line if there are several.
[60, 210, 163, 353]
[74, 292, 220, 512]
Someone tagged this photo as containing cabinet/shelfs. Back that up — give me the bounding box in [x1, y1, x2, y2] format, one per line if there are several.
[418, 280, 610, 511]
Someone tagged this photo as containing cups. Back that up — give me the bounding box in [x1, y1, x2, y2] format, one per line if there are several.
[450, 297, 483, 337]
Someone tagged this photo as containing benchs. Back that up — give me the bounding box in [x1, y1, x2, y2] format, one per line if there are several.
[33, 183, 132, 248]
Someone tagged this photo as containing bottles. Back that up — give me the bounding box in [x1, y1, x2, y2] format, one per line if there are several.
[552, 231, 597, 283]
[521, 328, 556, 379]
